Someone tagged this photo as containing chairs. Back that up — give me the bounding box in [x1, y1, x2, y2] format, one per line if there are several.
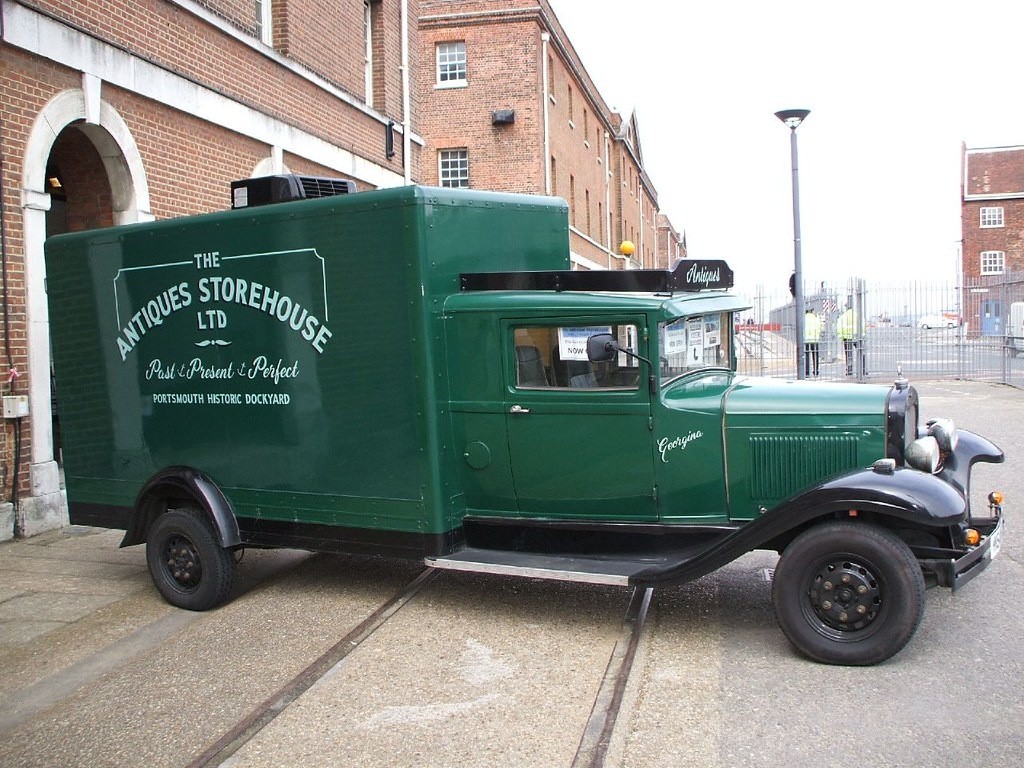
[514, 345, 551, 388]
[554, 344, 599, 389]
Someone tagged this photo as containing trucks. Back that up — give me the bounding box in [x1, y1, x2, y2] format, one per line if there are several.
[42, 183, 1009, 667]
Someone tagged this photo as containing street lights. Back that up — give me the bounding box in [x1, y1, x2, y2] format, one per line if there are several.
[774, 108, 814, 382]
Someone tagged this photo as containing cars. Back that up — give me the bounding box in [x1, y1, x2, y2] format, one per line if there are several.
[866, 312, 963, 330]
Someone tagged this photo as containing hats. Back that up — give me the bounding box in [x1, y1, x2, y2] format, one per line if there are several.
[806, 306, 814, 311]
[845, 302, 852, 309]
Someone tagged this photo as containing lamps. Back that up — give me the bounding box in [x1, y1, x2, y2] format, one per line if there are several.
[493, 111, 515, 124]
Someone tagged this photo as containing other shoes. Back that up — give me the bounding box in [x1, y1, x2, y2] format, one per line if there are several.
[806, 374, 818, 377]
[846, 372, 868, 376]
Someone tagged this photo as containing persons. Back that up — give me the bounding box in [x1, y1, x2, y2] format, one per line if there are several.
[805, 307, 820, 378]
[748, 317, 754, 324]
[837, 302, 868, 375]
